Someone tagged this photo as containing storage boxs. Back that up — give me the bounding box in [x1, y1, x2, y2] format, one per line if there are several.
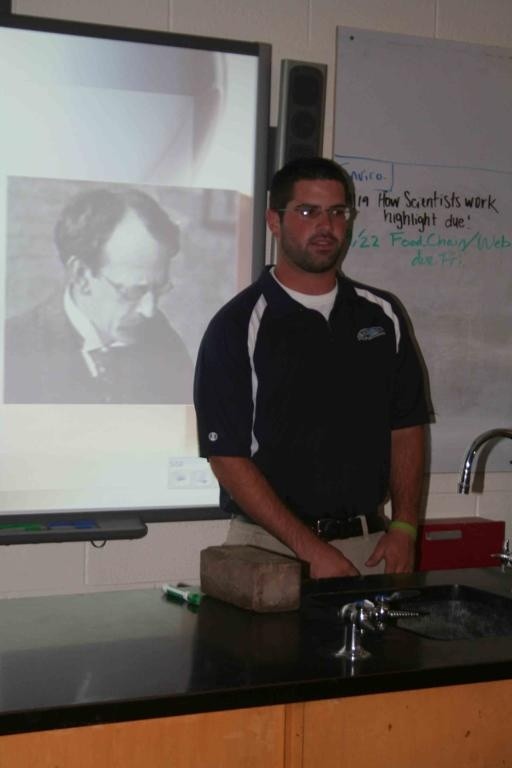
[416, 517, 505, 571]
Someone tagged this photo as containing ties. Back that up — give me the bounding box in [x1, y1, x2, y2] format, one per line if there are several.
[91, 348, 125, 401]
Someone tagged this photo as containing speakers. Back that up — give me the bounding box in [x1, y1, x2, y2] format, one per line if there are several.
[270, 57, 330, 171]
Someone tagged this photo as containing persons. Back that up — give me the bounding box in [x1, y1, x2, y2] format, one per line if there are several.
[7, 187, 194, 404]
[193, 155, 431, 580]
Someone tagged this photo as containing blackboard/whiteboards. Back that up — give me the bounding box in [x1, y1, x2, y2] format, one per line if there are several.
[330, 27, 512, 476]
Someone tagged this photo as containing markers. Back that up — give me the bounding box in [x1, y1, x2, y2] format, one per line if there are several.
[161, 582, 202, 607]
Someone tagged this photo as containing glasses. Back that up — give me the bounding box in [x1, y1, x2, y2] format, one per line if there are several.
[278, 205, 355, 223]
[98, 270, 176, 301]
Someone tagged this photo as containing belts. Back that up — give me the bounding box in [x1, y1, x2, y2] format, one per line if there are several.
[304, 513, 391, 540]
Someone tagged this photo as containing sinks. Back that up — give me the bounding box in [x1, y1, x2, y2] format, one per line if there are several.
[312, 582, 511, 642]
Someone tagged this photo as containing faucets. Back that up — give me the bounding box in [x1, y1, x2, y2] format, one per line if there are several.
[459, 426, 511, 574]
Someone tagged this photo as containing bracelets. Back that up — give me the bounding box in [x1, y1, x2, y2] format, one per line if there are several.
[387, 520, 419, 541]
